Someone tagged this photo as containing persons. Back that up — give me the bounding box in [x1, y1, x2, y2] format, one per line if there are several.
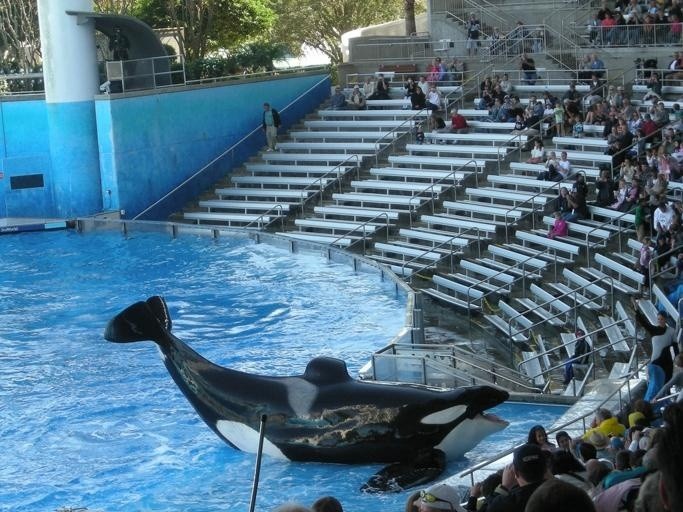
[105, 24, 130, 63]
[562, 330, 591, 385]
[326, 1, 683, 291]
[407, 400, 683, 511]
[260, 100, 283, 153]
[312, 497, 342, 510]
[630, 297, 681, 395]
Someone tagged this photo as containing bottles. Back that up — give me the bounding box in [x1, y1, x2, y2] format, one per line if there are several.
[420, 483, 468, 512]
[512, 444, 545, 473]
[588, 432, 610, 450]
[611, 437, 623, 449]
[575, 329, 586, 336]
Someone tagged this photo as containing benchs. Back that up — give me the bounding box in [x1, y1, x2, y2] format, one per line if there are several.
[183, 84, 433, 251]
[349, 83, 682, 398]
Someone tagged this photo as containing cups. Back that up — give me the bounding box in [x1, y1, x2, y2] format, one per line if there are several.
[418, 490, 453, 509]
